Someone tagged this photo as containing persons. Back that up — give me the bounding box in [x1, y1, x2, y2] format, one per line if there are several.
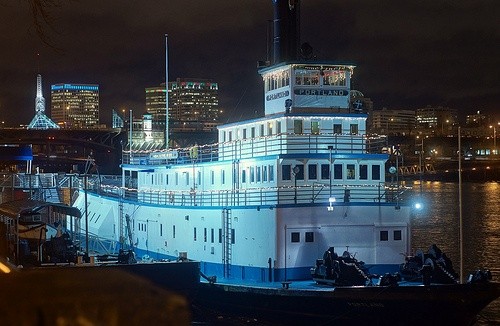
[118, 249, 126, 263]
[127, 250, 135, 265]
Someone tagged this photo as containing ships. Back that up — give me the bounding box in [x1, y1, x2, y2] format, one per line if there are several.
[12, 0, 500, 325]
